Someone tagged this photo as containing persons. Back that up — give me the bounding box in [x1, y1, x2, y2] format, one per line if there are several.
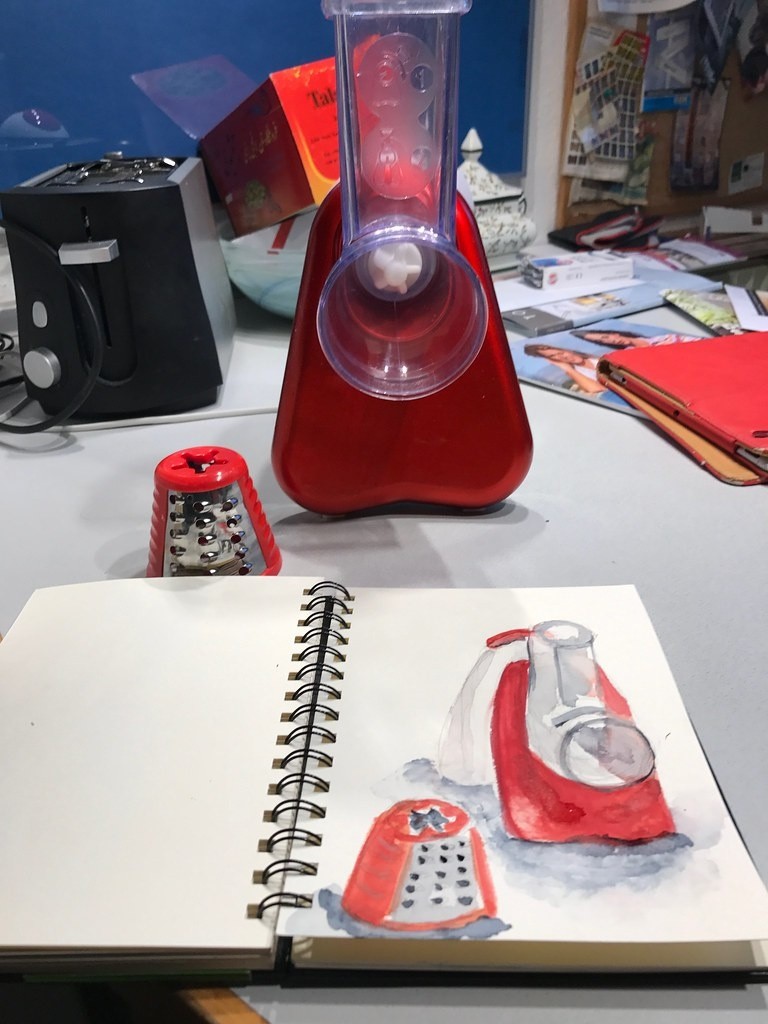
[524, 345, 609, 393]
[569, 329, 705, 351]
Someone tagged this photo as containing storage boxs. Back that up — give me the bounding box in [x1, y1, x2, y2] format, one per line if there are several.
[201, 32, 422, 240]
[520, 248, 633, 289]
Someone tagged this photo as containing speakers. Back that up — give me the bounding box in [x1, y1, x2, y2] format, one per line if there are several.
[0, 158, 239, 424]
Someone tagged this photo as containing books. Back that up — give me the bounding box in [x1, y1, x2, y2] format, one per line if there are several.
[497, 232, 768, 422]
[0, 576, 768, 990]
